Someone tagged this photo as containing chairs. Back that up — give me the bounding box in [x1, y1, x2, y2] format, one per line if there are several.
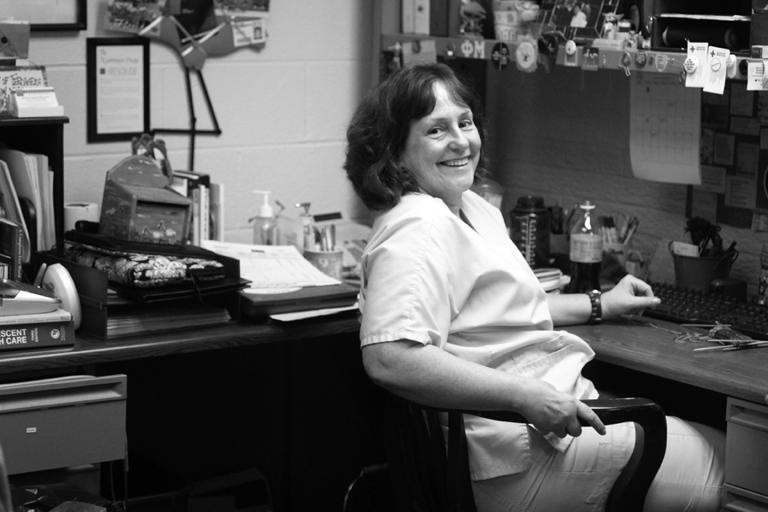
[338, 331, 666, 512]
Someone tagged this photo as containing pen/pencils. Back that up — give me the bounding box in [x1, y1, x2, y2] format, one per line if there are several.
[304, 224, 335, 251]
[597, 214, 639, 245]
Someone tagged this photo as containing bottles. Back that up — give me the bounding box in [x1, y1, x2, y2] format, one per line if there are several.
[758, 242, 768, 305]
[510, 196, 551, 272]
[569, 200, 603, 293]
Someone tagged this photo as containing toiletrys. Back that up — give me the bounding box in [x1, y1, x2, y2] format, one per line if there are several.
[297, 201, 315, 251]
[253, 190, 276, 246]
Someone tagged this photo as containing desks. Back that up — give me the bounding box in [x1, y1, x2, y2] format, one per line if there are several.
[0, 316, 357, 512]
[553, 315, 768, 512]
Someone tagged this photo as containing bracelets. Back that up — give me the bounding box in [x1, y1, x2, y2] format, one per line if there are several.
[586, 290, 604, 325]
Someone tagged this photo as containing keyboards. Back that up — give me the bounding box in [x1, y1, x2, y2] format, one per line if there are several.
[644, 280, 768, 340]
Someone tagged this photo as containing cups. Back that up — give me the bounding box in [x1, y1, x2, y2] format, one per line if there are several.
[602, 243, 644, 282]
[304, 248, 344, 285]
[493, 10, 525, 46]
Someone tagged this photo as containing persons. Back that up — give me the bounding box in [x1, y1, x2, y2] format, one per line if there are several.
[341, 63, 728, 512]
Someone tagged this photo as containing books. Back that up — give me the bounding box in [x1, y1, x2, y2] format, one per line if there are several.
[24, 257, 231, 341]
[0, 21, 75, 355]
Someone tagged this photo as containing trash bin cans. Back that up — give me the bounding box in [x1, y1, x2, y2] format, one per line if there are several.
[20, 489, 122, 512]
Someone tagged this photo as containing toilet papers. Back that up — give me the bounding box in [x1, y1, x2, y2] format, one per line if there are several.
[64, 202, 96, 230]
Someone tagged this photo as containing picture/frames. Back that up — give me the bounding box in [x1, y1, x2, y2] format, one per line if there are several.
[87, 36, 150, 143]
[0, 0, 87, 31]
